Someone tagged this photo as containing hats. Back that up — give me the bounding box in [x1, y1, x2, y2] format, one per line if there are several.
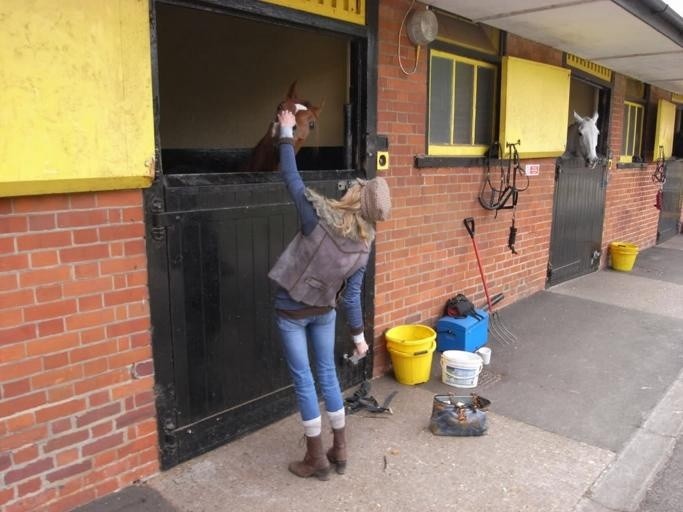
[356, 176, 391, 221]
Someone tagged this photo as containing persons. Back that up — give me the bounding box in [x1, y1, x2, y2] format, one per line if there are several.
[265, 108, 392, 482]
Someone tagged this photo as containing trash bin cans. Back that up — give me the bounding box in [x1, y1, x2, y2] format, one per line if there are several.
[609, 242, 639, 272]
[384, 324, 436, 385]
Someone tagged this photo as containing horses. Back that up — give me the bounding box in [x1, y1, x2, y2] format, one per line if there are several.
[562, 111, 600, 169]
[238, 78, 326, 172]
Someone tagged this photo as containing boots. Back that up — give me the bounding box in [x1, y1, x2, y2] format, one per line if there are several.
[288, 433, 331, 481]
[328, 428, 347, 475]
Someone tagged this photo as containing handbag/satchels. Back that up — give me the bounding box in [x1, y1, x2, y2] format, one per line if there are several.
[430, 392, 491, 436]
[446, 294, 474, 319]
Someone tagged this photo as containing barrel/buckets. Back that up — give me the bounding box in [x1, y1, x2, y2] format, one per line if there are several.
[441, 350, 485, 389]
[610, 241, 640, 272]
[385, 322, 437, 387]
